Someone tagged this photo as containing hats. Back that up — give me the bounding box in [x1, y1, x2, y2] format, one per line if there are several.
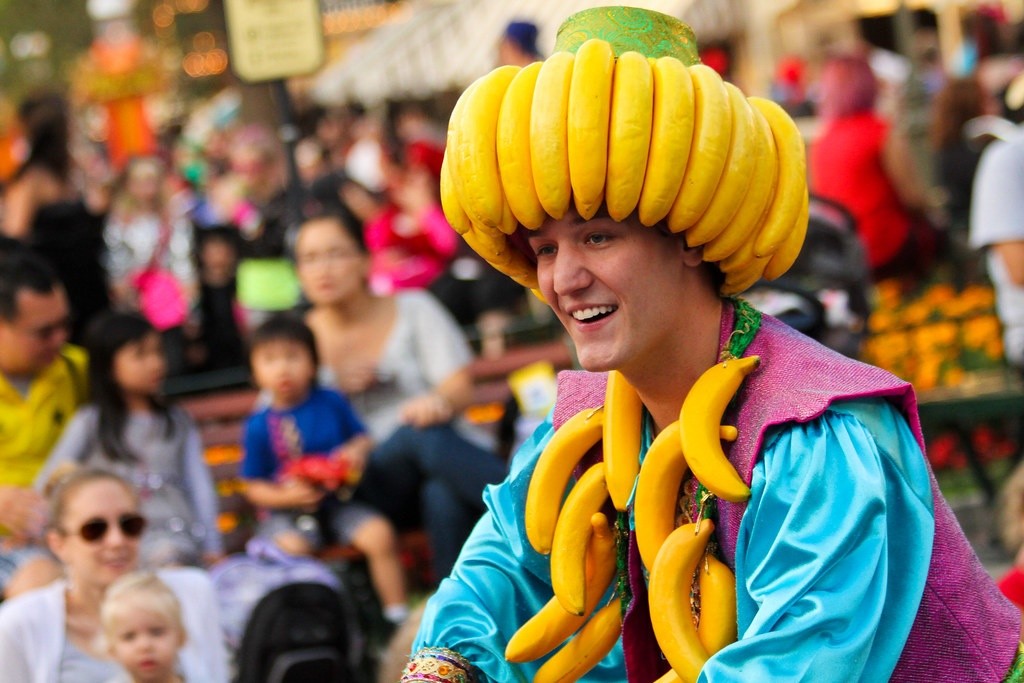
[439, 6, 814, 297]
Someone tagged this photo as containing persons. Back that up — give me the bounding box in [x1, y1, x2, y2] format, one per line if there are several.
[0, 462, 229, 683]
[0, 88, 478, 363]
[496, 19, 545, 66]
[238, 317, 409, 632]
[0, 234, 91, 548]
[966, 123, 1024, 369]
[804, 56, 954, 280]
[293, 213, 504, 590]
[96, 573, 189, 683]
[932, 81, 1002, 226]
[33, 313, 227, 570]
[399, 6, 1024, 683]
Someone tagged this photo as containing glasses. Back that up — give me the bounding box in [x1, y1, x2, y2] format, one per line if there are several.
[5, 309, 77, 341]
[53, 515, 145, 543]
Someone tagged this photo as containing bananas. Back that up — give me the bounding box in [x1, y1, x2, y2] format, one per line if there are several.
[435, 42, 810, 298]
[502, 354, 760, 682]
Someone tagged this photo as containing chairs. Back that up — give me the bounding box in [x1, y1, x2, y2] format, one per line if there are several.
[172, 394, 426, 563]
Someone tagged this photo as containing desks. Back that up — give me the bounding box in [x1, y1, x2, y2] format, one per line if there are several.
[918, 377, 1024, 559]
